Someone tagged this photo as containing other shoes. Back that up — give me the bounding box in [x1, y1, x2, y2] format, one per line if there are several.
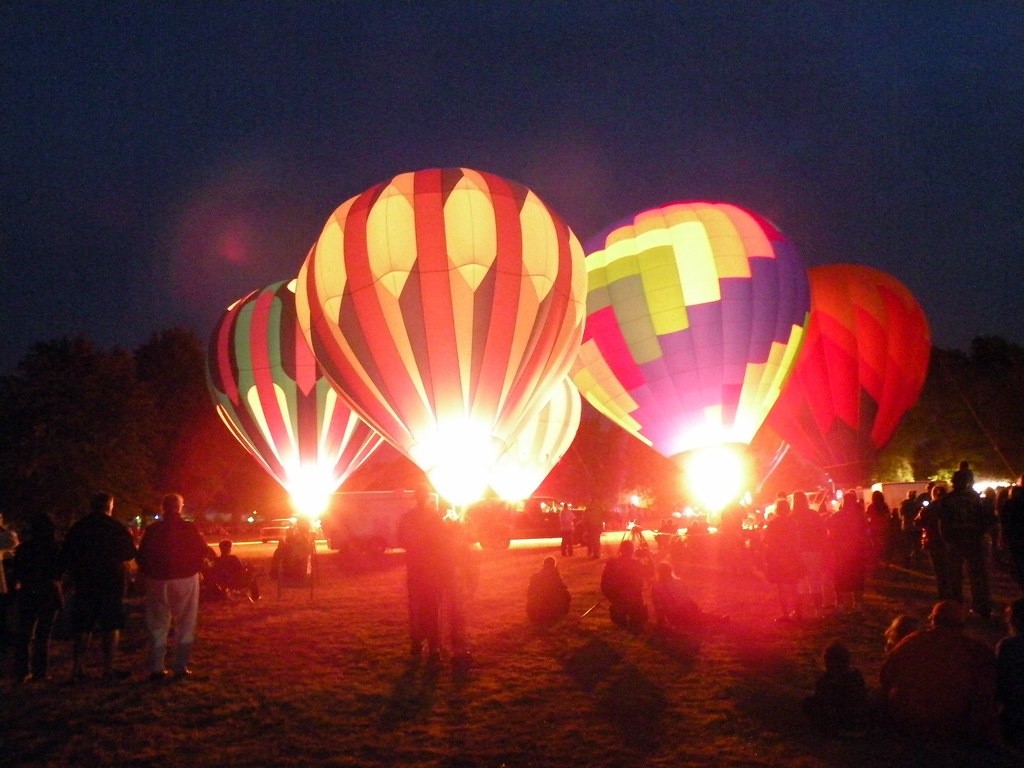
[176, 666, 193, 680]
[24, 674, 48, 694]
[150, 671, 166, 684]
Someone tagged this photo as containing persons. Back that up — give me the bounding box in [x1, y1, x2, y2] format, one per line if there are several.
[555, 461, 1024, 734]
[0, 482, 255, 703]
[525, 556, 573, 626]
[397, 484, 483, 679]
[136, 492, 209, 680]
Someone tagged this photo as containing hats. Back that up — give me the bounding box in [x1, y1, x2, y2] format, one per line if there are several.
[928, 600, 968, 623]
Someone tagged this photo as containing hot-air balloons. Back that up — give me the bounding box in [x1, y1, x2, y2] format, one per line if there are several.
[295, 169, 588, 511]
[206, 276, 386, 532]
[762, 263, 930, 500]
[564, 200, 814, 520]
[490, 374, 581, 510]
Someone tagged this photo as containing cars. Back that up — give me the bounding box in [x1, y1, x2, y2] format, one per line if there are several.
[261, 519, 295, 544]
[474, 498, 607, 553]
